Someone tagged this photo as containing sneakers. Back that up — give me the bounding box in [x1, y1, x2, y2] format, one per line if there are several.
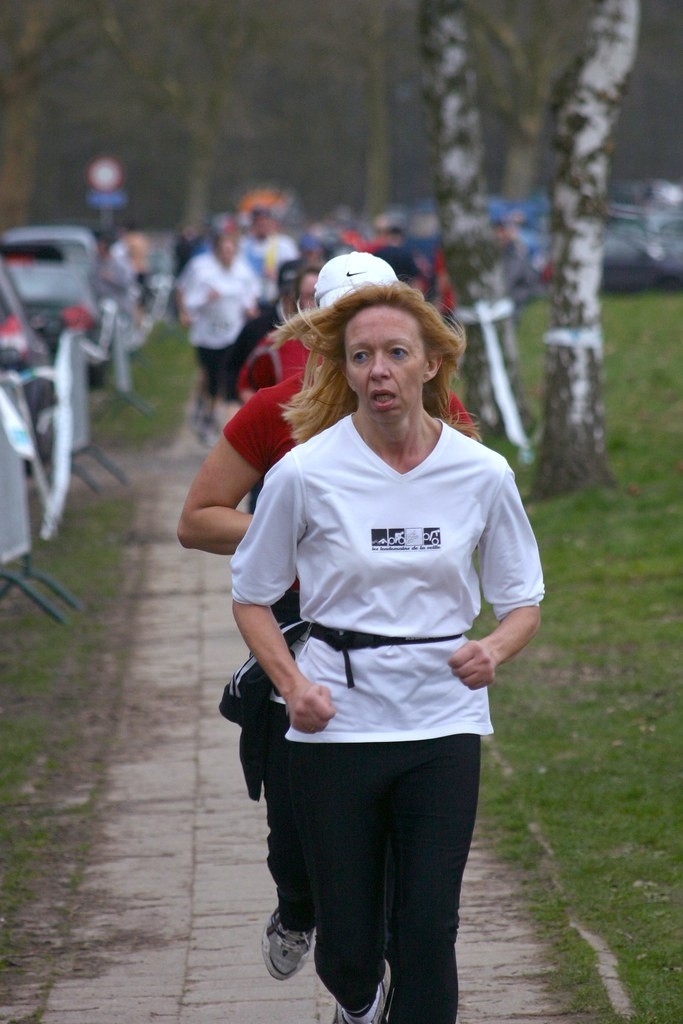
[262, 904, 315, 981]
[332, 959, 391, 1024]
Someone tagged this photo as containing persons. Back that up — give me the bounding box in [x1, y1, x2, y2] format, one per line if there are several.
[90, 206, 683, 1024]
[229, 284, 545, 1024]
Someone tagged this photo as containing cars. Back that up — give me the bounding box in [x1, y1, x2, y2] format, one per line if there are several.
[599, 179, 683, 290]
[0, 222, 130, 469]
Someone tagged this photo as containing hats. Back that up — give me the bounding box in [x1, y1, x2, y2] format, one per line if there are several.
[314, 250, 399, 310]
[372, 244, 424, 284]
[277, 257, 310, 291]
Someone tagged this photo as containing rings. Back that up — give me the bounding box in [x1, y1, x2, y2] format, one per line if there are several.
[306, 723, 317, 733]
